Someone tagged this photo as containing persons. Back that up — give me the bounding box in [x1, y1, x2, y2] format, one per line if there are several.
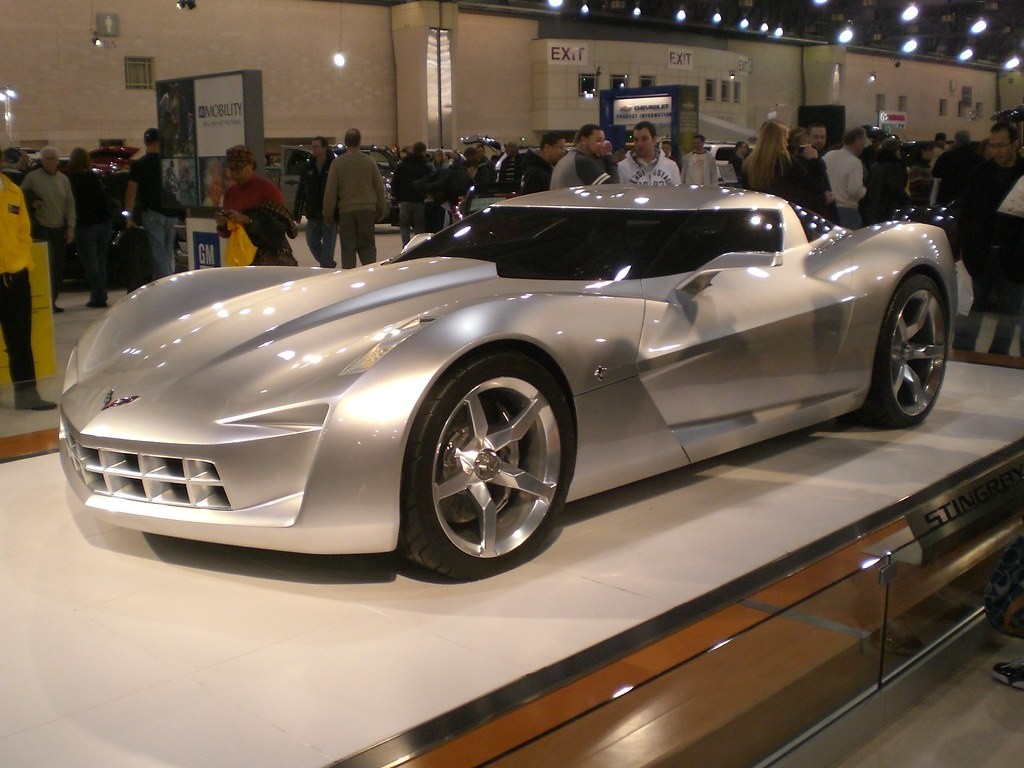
[391, 141, 437, 248]
[497, 142, 520, 191]
[464, 163, 493, 217]
[426, 159, 478, 233]
[400, 149, 407, 159]
[518, 132, 566, 196]
[433, 150, 449, 171]
[0, 173, 56, 410]
[69, 147, 112, 307]
[0, 148, 23, 185]
[215, 144, 297, 266]
[20, 146, 76, 313]
[617, 104, 1024, 313]
[124, 127, 178, 280]
[451, 149, 461, 164]
[475, 145, 488, 165]
[291, 137, 337, 268]
[18, 149, 30, 171]
[550, 124, 620, 191]
[324, 128, 385, 269]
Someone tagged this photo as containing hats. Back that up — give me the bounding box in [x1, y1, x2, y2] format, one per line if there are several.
[226, 144, 255, 161]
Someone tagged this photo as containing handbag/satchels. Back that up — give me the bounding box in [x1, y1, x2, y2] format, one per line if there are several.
[430, 169, 467, 211]
[223, 217, 258, 266]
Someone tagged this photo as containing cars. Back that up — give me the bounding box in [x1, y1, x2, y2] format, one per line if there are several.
[461, 132, 575, 185]
[276, 142, 409, 227]
[424, 148, 467, 171]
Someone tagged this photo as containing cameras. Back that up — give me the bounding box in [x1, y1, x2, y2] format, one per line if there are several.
[799, 145, 806, 153]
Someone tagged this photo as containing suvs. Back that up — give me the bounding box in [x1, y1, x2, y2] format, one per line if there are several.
[701, 140, 763, 188]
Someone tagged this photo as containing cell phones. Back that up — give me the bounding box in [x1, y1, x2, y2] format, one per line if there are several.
[218, 208, 229, 218]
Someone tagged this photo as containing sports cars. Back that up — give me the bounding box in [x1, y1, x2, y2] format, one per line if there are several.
[58, 179, 959, 578]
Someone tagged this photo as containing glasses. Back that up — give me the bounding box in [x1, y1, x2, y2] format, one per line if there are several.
[225, 163, 248, 172]
[989, 140, 1013, 150]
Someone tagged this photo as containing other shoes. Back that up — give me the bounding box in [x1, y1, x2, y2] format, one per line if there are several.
[87, 301, 108, 307]
[16, 399, 57, 410]
[53, 306, 64, 312]
